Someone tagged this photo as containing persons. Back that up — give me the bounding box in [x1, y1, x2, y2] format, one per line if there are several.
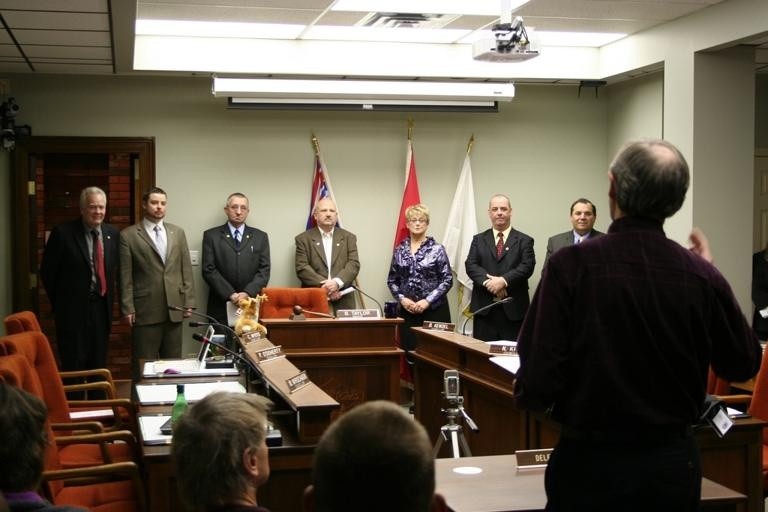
[294, 197, 362, 316]
[0, 382, 91, 511]
[463, 192, 537, 343]
[751, 247, 768, 344]
[38, 183, 121, 402]
[199, 192, 272, 335]
[511, 139, 764, 511]
[169, 391, 273, 512]
[541, 197, 609, 275]
[385, 203, 455, 415]
[118, 185, 199, 398]
[298, 398, 446, 511]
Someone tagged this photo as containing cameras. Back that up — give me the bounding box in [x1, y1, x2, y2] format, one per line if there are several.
[438, 365, 474, 425]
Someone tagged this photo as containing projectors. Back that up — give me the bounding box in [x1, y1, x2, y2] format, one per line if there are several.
[473, 15, 540, 65]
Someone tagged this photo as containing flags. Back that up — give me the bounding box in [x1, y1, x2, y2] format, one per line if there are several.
[305, 153, 343, 231]
[440, 151, 480, 338]
[393, 142, 421, 251]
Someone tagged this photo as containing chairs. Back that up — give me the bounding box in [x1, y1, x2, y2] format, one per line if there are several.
[261, 288, 330, 318]
[707, 341, 768, 500]
[0, 309, 146, 511]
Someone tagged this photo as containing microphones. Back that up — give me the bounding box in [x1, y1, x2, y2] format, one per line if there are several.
[463, 296, 518, 335]
[192, 332, 263, 375]
[350, 285, 386, 318]
[168, 304, 228, 334]
[190, 319, 248, 349]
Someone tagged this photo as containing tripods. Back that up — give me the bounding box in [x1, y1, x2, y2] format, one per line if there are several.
[430, 421, 475, 458]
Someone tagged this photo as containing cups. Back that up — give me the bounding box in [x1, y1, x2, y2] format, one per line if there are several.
[205, 334, 226, 361]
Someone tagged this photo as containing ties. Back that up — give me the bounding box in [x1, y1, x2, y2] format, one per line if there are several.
[91, 229, 107, 297]
[154, 225, 166, 263]
[497, 233, 504, 259]
[233, 230, 241, 247]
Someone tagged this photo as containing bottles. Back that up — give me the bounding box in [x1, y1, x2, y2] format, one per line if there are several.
[171, 383, 191, 433]
